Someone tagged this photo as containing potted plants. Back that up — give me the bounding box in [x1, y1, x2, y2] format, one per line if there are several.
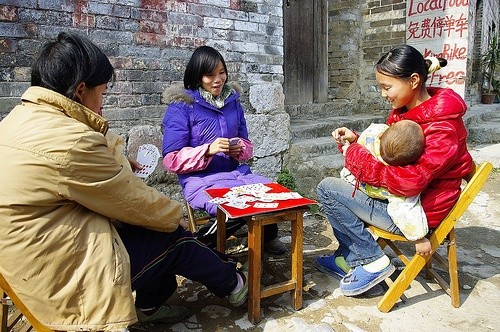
[478, 32, 500, 104]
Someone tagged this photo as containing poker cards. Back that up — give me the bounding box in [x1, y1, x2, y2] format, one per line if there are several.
[229, 138, 239, 146]
[339, 134, 347, 146]
[133, 144, 160, 178]
[209, 182, 304, 211]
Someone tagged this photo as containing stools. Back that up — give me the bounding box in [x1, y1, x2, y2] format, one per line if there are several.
[216, 204, 311, 324]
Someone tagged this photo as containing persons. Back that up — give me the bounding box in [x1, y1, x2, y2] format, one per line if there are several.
[0, 31, 263, 332]
[162, 46, 287, 255]
[314, 45, 472, 297]
[339, 120, 432, 257]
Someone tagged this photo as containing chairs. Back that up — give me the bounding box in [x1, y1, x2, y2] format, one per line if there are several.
[370, 162, 495, 313]
[0, 272, 56, 332]
[186, 197, 249, 241]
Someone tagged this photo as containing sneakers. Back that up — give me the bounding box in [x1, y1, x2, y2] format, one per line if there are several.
[229, 257, 262, 307]
[137, 305, 187, 322]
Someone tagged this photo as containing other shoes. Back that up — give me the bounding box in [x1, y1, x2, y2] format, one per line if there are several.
[263, 237, 288, 254]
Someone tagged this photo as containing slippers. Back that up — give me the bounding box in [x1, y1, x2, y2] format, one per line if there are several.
[340, 261, 396, 296]
[315, 252, 347, 281]
